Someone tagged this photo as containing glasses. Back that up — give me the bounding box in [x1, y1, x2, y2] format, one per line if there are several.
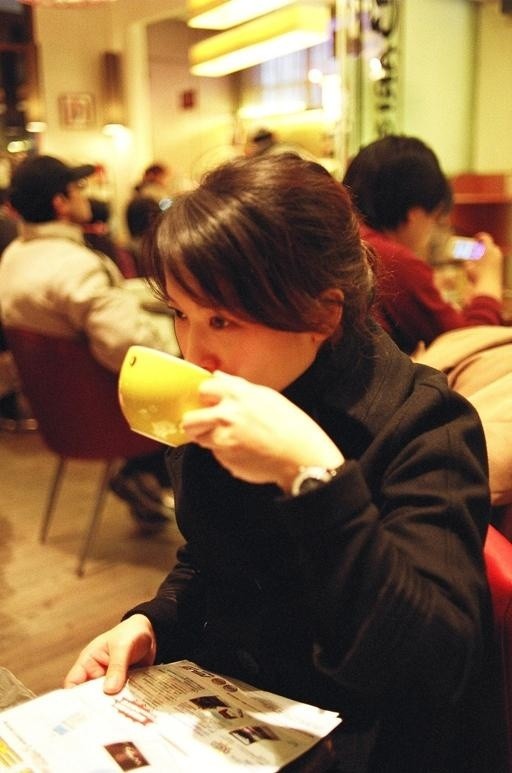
[76, 176, 88, 189]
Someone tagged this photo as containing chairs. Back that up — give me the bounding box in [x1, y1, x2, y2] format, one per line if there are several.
[484, 521, 512, 759]
[2, 325, 161, 577]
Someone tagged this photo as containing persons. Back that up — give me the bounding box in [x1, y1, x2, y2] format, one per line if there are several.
[1, 130, 512, 524]
[64, 151, 512, 772]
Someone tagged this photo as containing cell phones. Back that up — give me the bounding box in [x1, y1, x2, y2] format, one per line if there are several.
[444, 235, 485, 262]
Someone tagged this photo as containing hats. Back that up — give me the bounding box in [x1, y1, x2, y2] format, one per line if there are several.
[10, 157, 94, 202]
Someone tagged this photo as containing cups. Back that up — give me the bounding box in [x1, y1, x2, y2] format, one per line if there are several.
[118, 345, 219, 452]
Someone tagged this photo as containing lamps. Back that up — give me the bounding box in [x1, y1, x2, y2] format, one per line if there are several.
[186, 0, 332, 80]
